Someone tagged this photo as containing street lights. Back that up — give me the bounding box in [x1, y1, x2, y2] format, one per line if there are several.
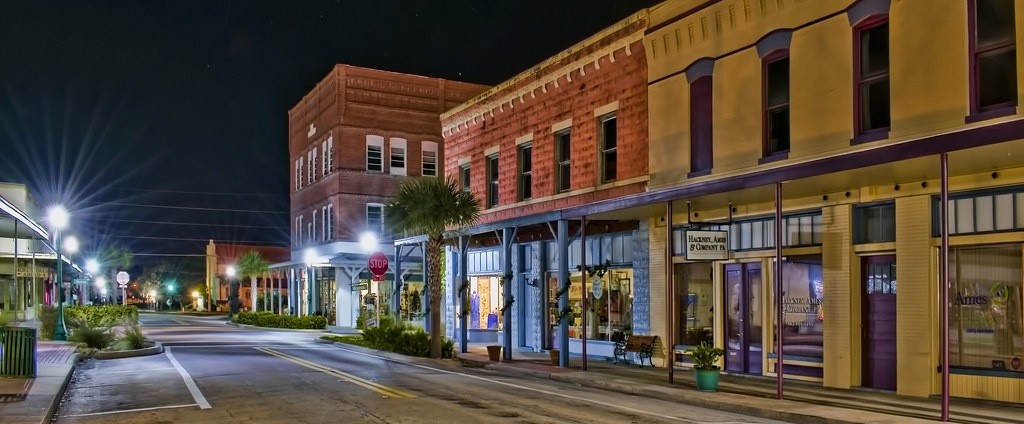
[226, 266, 240, 314]
[168, 285, 173, 312]
[47, 204, 71, 340]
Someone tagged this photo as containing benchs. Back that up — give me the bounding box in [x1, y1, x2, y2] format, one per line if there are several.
[615, 334, 657, 368]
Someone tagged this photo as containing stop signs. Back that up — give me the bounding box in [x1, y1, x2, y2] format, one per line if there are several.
[368, 252, 388, 276]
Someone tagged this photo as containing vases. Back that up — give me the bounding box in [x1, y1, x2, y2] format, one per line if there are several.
[549, 350, 558, 366]
[487, 346, 503, 361]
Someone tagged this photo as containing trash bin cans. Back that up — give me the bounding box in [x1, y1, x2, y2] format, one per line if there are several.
[0, 326, 37, 378]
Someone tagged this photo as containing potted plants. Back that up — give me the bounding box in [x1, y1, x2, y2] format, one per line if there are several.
[685, 342, 725, 394]
